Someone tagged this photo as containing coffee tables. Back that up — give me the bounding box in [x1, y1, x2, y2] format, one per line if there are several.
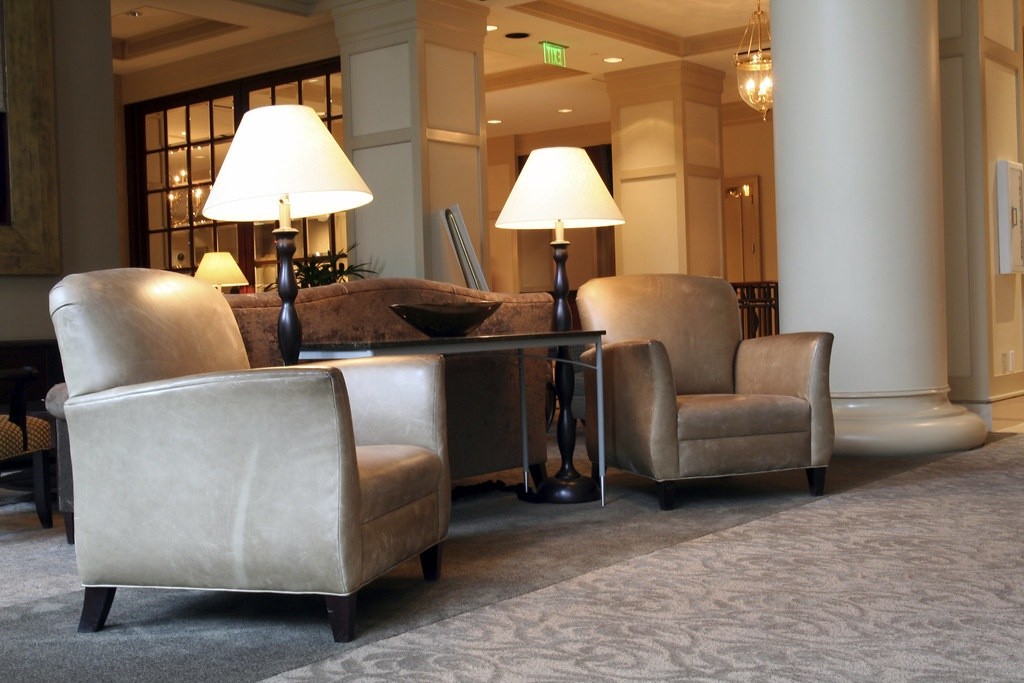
[285, 329, 608, 507]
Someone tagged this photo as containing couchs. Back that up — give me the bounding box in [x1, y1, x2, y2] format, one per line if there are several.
[0, 414, 55, 526]
[214, 278, 556, 494]
[46, 267, 454, 645]
[575, 275, 835, 512]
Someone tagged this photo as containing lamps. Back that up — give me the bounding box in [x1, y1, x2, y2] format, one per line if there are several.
[493, 146, 626, 504]
[733, 1, 775, 123]
[202, 104, 375, 371]
